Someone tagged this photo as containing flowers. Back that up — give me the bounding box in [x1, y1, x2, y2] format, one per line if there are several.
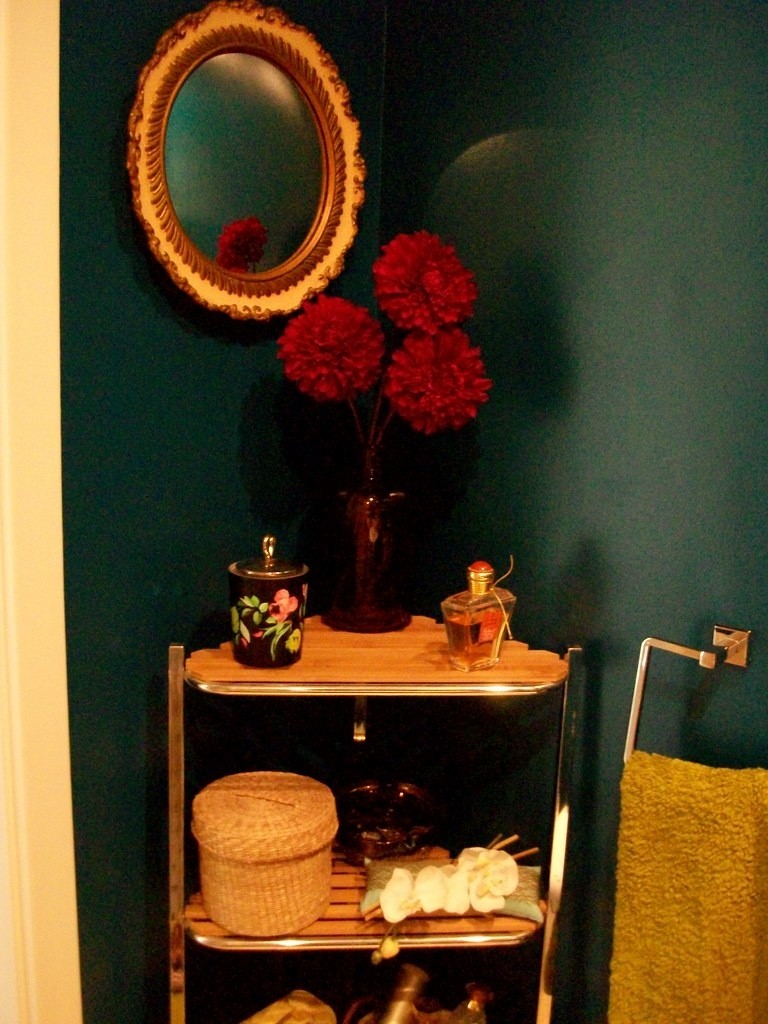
[213, 218, 268, 277]
[371, 832, 540, 966]
[274, 231, 496, 491]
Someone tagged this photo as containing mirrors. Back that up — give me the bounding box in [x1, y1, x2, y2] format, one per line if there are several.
[128, 0, 366, 323]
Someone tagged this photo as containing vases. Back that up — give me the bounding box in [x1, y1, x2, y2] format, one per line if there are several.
[326, 490, 414, 633]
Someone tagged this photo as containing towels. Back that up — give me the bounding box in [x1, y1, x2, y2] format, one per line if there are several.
[608, 750, 768, 1024]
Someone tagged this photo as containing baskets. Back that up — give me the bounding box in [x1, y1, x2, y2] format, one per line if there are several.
[192, 772, 338, 937]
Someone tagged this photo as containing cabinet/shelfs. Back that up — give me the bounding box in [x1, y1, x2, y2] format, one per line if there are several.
[166, 613, 587, 1024]
